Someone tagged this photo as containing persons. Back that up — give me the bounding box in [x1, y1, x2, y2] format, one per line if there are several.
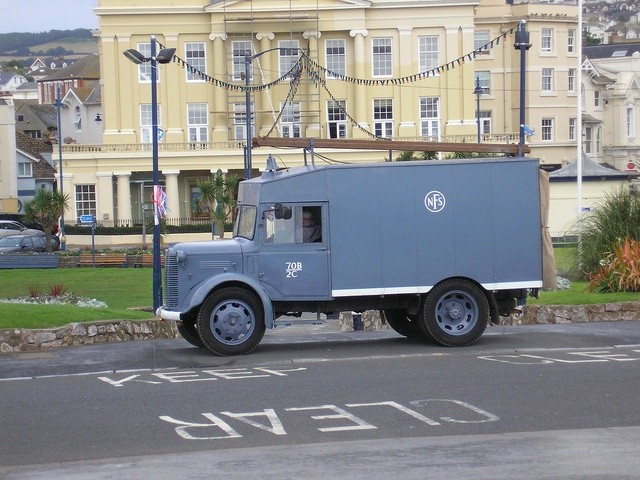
[285, 210, 322, 243]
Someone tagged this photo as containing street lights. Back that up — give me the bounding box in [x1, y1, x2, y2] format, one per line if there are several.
[472, 77, 484, 143]
[515, 18, 533, 144]
[123, 38, 177, 315]
[50, 84, 67, 250]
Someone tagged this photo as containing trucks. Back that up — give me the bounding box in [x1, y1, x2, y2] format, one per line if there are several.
[157, 154, 558, 356]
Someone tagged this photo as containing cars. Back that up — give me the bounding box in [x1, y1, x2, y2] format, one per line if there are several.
[0, 213, 60, 256]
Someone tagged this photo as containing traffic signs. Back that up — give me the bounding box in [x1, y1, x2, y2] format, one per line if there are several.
[79, 215, 93, 223]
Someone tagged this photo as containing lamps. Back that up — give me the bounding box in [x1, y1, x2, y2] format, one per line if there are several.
[95, 113, 102, 121]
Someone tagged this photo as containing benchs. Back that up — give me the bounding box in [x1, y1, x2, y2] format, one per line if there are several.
[134, 254, 166, 268]
[76, 253, 128, 268]
